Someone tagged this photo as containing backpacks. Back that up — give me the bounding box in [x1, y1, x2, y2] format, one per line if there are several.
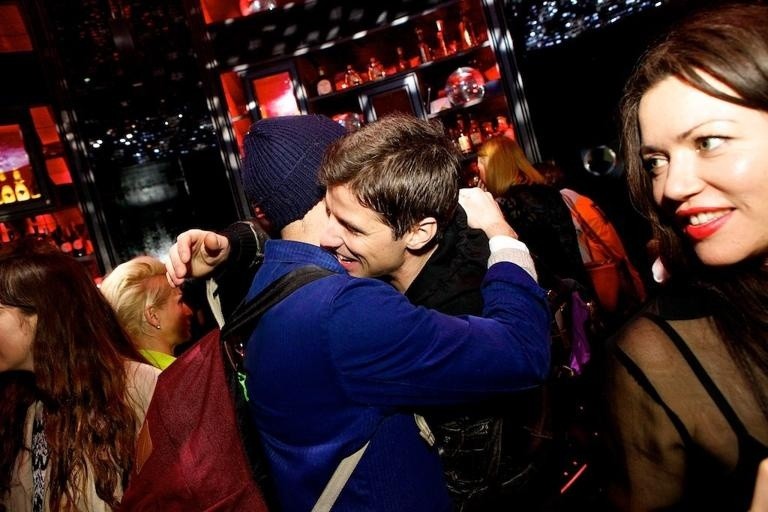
[559, 183, 647, 314]
[123, 265, 334, 511]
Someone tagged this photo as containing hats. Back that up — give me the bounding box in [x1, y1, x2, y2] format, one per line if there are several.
[244, 115, 349, 235]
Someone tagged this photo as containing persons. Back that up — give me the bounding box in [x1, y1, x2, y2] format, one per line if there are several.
[476, 133, 612, 378]
[612, 3, 768, 511]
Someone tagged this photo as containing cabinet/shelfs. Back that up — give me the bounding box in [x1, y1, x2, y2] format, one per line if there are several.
[197, 0, 540, 224]
[0, 54, 117, 288]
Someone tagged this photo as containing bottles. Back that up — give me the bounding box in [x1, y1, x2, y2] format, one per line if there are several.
[1, 168, 86, 259]
[313, 10, 508, 154]
